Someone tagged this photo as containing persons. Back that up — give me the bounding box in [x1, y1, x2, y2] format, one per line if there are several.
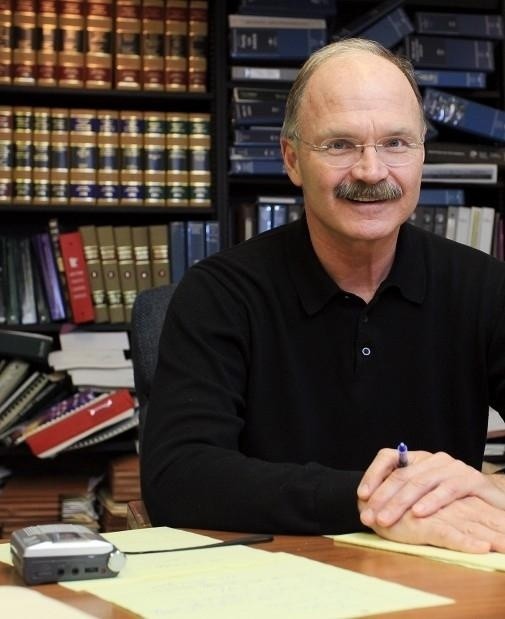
[138, 37, 505, 554]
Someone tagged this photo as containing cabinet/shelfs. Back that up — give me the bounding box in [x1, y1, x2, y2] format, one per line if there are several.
[1, 0, 505, 538]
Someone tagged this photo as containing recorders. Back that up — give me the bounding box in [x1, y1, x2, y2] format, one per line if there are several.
[11, 524, 128, 584]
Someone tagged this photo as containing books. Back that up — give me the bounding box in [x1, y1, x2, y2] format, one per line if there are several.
[403, 205, 504, 262]
[0, 288, 153, 544]
[1, 2, 213, 207]
[0, 217, 219, 325]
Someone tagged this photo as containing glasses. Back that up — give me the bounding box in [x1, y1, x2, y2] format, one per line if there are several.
[296, 132, 425, 167]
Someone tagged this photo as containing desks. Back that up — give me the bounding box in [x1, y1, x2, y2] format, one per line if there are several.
[0, 525, 505, 619]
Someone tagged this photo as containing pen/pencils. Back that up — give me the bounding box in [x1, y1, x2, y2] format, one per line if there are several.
[397, 442, 409, 467]
